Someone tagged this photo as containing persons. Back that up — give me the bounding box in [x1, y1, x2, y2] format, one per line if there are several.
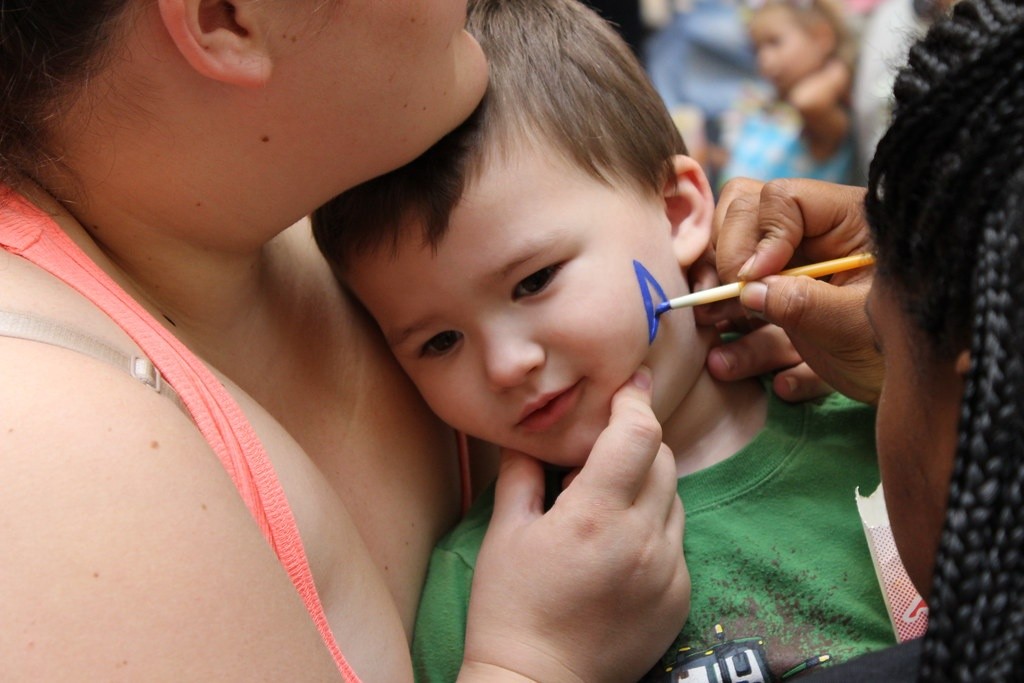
[0, 2, 843, 683]
[309, 1, 949, 683]
[862, 2, 952, 180]
[706, 2, 856, 188]
[689, 4, 1023, 683]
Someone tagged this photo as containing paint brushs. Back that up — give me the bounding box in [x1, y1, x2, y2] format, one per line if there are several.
[652, 252, 879, 318]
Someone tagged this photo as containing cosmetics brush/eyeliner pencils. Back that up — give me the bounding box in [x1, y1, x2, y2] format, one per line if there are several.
[656, 249, 881, 319]
